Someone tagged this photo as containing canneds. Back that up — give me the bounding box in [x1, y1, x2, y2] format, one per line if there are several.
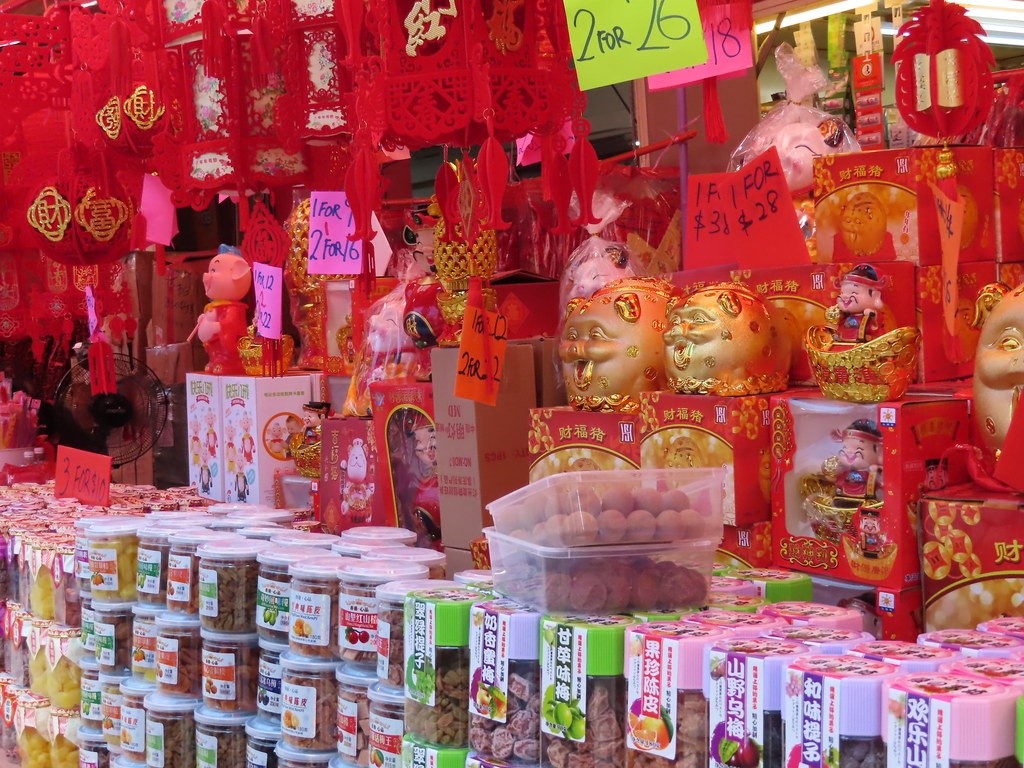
[0, 478, 321, 768]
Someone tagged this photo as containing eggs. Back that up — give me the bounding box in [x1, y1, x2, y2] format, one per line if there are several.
[489, 485, 703, 550]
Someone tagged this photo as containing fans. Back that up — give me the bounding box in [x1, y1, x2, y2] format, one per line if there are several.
[53, 353, 170, 469]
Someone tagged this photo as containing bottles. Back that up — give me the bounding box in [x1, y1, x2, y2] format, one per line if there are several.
[155, 392, 173, 446]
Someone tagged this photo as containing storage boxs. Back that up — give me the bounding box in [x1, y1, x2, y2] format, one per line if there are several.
[431, 345, 536, 546]
[484, 272, 560, 350]
[769, 392, 957, 592]
[487, 467, 729, 546]
[915, 481, 1024, 633]
[636, 394, 773, 507]
[322, 275, 400, 375]
[814, 145, 997, 263]
[318, 419, 398, 528]
[371, 379, 442, 556]
[993, 148, 1023, 261]
[483, 525, 719, 615]
[96, 253, 312, 521]
[731, 261, 913, 381]
[919, 259, 998, 384]
[529, 408, 638, 490]
